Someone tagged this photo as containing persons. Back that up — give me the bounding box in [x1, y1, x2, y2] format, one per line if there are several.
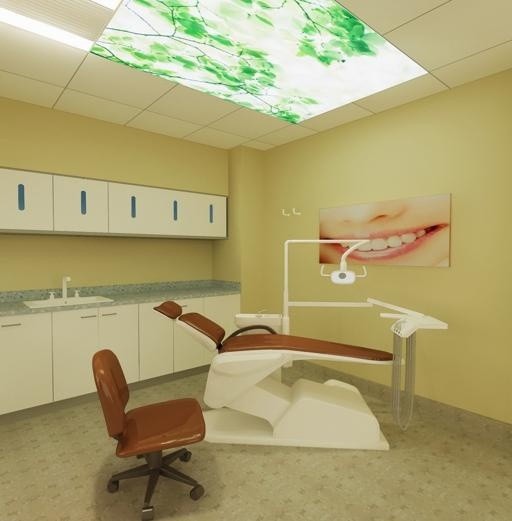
[319, 193, 451, 267]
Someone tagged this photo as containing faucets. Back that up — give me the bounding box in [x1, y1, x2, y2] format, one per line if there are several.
[62, 276, 70, 298]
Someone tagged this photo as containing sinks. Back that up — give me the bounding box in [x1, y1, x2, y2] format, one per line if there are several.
[23, 296, 114, 310]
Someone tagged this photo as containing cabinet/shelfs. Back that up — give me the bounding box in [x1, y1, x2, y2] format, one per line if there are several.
[1, 166, 228, 241]
[1, 294, 240, 427]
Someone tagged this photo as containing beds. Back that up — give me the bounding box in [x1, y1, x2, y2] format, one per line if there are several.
[152, 300, 404, 452]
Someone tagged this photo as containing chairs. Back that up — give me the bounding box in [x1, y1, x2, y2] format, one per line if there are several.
[92, 349, 206, 521]
